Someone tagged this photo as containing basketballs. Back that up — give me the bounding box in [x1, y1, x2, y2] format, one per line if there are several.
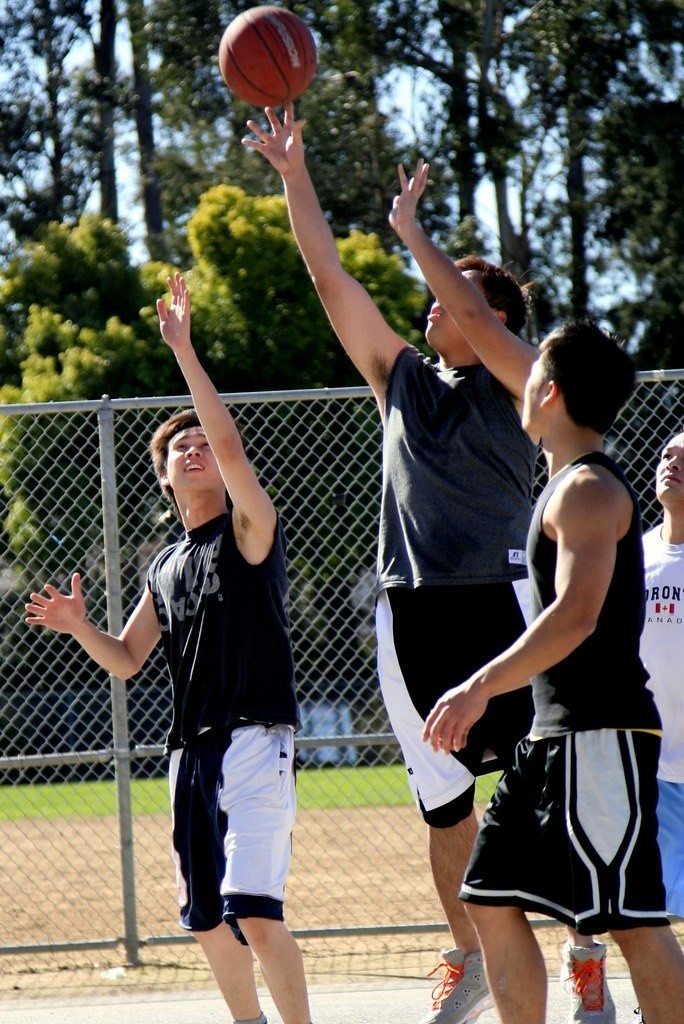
[217, 3, 319, 108]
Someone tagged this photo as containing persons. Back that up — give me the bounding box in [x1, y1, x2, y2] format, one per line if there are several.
[634, 432, 684, 1023]
[388, 160, 684, 1024]
[241, 102, 616, 1024]
[25, 272, 313, 1024]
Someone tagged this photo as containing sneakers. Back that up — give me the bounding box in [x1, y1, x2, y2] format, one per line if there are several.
[559, 939, 617, 1024]
[422, 947, 496, 1024]
